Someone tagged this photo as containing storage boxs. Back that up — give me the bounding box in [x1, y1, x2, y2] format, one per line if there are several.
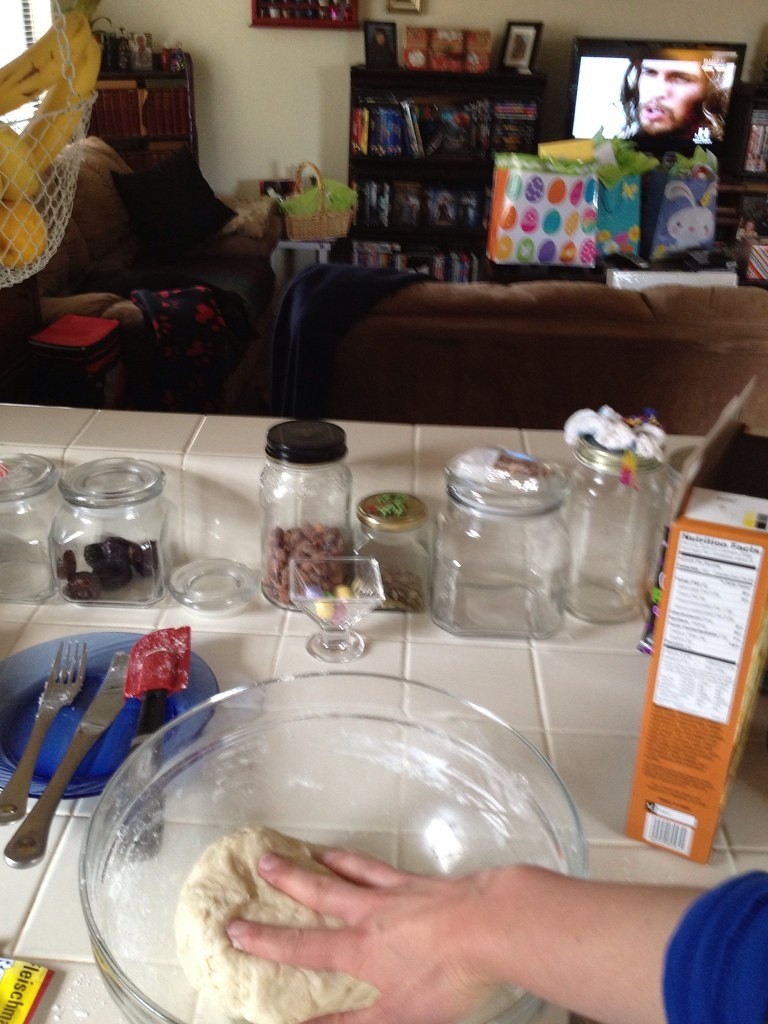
[623, 375, 768, 864]
[401, 26, 495, 75]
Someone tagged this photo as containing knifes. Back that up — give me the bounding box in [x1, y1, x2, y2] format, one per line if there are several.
[5, 650, 136, 870]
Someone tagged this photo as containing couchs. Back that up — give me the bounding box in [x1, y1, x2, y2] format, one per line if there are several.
[284, 265, 768, 436]
[38, 129, 280, 395]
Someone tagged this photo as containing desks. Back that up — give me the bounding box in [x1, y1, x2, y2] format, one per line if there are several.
[0, 397, 768, 1024]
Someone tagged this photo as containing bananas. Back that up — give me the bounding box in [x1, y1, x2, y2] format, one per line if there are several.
[0, 120, 42, 201]
[0, 9, 102, 180]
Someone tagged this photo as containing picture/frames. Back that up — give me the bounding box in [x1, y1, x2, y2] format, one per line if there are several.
[362, 19, 397, 68]
[386, 0, 423, 16]
[500, 19, 547, 70]
[251, 0, 361, 29]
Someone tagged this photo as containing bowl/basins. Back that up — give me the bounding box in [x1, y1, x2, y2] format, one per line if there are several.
[77, 669, 584, 1023]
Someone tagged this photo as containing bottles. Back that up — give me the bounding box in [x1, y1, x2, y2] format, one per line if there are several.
[429, 449, 569, 638]
[0, 451, 74, 600]
[560, 419, 673, 627]
[47, 455, 177, 609]
[259, 422, 355, 614]
[357, 493, 437, 617]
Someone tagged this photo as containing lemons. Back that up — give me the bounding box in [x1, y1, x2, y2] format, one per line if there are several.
[0, 200, 49, 271]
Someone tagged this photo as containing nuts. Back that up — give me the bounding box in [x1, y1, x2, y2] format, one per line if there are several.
[265, 522, 346, 607]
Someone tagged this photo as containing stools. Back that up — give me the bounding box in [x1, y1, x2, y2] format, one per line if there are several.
[276, 238, 342, 264]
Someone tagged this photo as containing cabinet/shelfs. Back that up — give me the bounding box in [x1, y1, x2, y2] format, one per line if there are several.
[348, 65, 549, 280]
[96, 52, 200, 165]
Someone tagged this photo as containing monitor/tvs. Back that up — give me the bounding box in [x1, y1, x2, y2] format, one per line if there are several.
[564, 36, 747, 158]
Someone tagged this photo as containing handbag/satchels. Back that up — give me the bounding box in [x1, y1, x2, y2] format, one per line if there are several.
[485, 157, 719, 270]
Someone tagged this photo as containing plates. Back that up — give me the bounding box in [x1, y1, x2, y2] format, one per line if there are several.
[0, 630, 220, 799]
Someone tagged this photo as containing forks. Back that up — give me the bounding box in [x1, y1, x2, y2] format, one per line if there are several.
[1, 638, 88, 827]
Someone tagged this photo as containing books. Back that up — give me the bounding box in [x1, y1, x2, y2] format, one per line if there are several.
[352, 95, 538, 283]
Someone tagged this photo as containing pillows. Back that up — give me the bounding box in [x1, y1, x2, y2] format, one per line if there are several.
[111, 142, 240, 260]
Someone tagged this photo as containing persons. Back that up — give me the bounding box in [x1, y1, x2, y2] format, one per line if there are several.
[228, 848, 768, 1024]
[620, 58, 730, 142]
[370, 29, 393, 64]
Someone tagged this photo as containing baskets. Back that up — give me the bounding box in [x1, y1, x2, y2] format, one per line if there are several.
[0, 0, 99, 287]
[284, 162, 353, 241]
